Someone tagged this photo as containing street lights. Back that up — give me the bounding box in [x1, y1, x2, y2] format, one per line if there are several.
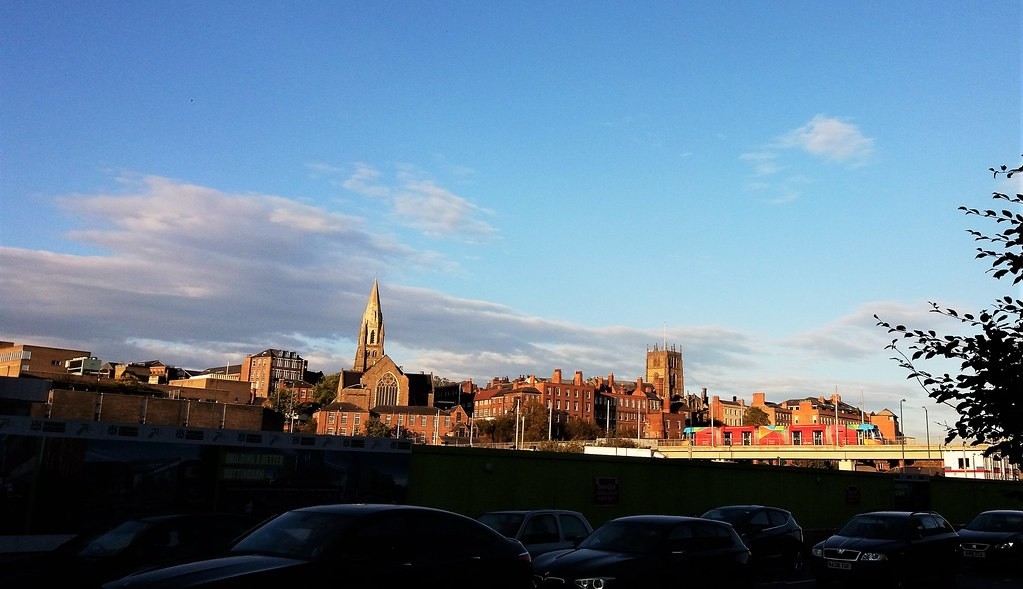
[900, 399, 906, 474]
[922, 406, 931, 475]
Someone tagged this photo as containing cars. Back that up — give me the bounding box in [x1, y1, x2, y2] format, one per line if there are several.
[960, 510, 1023, 581]
[476, 510, 601, 557]
[532, 515, 754, 589]
[101, 504, 533, 589]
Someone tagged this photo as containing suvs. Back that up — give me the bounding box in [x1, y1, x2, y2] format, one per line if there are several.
[808, 510, 960, 589]
[698, 505, 804, 582]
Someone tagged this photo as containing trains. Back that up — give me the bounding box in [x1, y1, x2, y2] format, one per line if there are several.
[682, 423, 884, 446]
